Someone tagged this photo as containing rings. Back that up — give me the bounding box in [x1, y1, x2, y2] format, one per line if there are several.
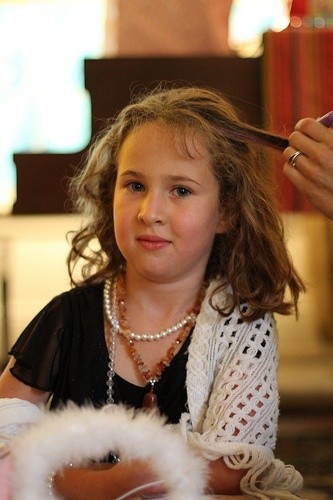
[287, 150, 303, 168]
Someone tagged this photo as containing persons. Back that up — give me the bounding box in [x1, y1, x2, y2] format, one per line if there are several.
[283, 117, 333, 221]
[0, 82, 304, 500]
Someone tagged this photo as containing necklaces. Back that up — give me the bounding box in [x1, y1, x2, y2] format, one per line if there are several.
[102, 265, 213, 416]
